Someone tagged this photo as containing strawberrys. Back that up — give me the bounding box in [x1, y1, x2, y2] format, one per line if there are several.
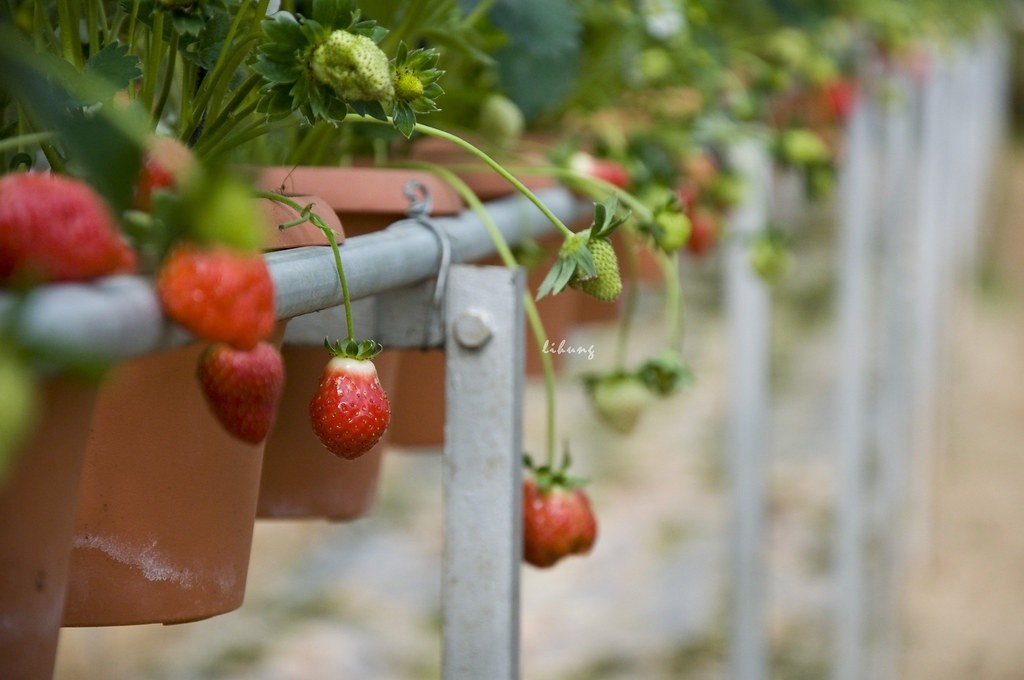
[0, 81, 855, 568]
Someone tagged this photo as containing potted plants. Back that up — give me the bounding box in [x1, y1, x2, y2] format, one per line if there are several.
[0, 0, 717, 680]
